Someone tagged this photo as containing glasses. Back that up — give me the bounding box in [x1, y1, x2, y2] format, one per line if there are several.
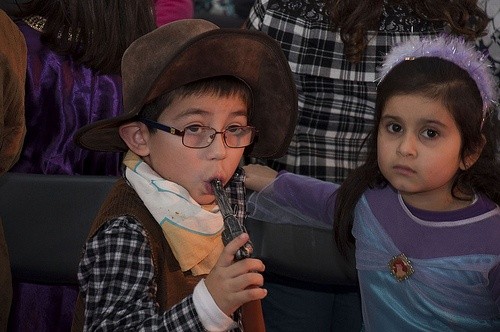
[147, 120, 257, 150]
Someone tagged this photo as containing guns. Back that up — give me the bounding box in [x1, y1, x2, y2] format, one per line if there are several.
[209, 178, 266, 332]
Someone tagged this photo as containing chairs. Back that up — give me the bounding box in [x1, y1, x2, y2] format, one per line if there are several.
[1, 173, 363, 332]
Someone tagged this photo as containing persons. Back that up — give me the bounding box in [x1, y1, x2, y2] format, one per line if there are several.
[10, 1, 157, 332]
[69, 19, 298, 332]
[241, 1, 500, 198]
[239, 36, 500, 332]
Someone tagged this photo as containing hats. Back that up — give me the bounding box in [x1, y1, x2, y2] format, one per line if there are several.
[72, 18, 297, 159]
